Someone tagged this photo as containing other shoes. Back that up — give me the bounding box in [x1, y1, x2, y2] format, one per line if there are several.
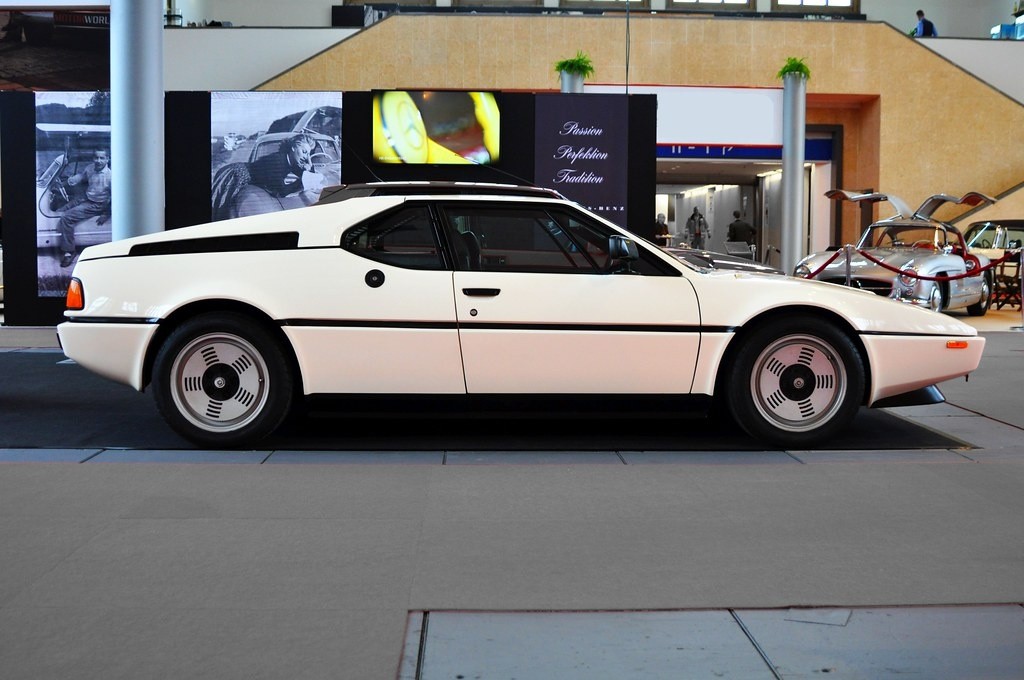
[61, 251, 76, 267]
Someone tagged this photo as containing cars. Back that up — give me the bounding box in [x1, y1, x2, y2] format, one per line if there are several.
[957, 220, 1024, 278]
[249, 111, 340, 175]
[55, 181, 986, 448]
[791, 189, 993, 316]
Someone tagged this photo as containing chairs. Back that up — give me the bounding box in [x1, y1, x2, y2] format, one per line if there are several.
[452, 229, 471, 270]
[915, 242, 934, 249]
[462, 231, 482, 270]
[989, 248, 1021, 310]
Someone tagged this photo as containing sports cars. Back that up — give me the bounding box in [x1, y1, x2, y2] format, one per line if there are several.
[35, 152, 112, 247]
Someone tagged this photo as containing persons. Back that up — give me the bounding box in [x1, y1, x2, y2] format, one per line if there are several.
[212, 134, 316, 220]
[728, 211, 757, 259]
[56, 146, 111, 267]
[915, 10, 938, 37]
[655, 214, 671, 246]
[684, 206, 711, 250]
